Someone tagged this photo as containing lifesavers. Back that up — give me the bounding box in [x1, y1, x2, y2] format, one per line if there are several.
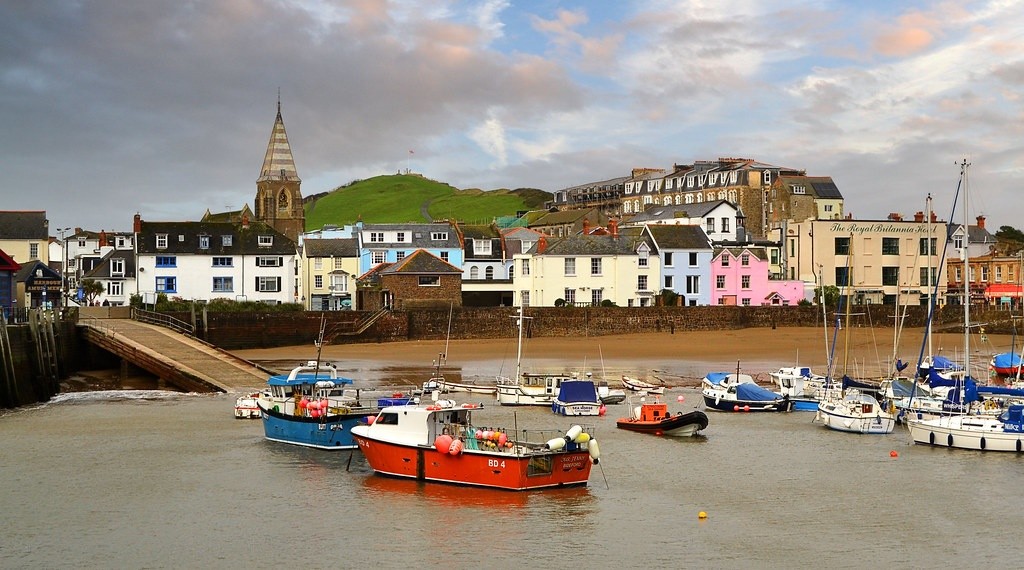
[426, 406, 442, 410]
[461, 403, 478, 408]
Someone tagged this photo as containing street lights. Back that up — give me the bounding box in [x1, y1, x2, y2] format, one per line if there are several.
[57, 228, 72, 289]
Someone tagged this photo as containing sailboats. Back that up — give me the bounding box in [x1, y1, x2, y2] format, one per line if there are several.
[769, 157, 1024, 453]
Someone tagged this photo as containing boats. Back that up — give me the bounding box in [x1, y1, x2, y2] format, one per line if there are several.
[268, 362, 352, 399]
[235, 391, 262, 419]
[701, 359, 797, 413]
[412, 292, 668, 417]
[617, 391, 709, 438]
[256, 311, 423, 451]
[350, 353, 593, 492]
